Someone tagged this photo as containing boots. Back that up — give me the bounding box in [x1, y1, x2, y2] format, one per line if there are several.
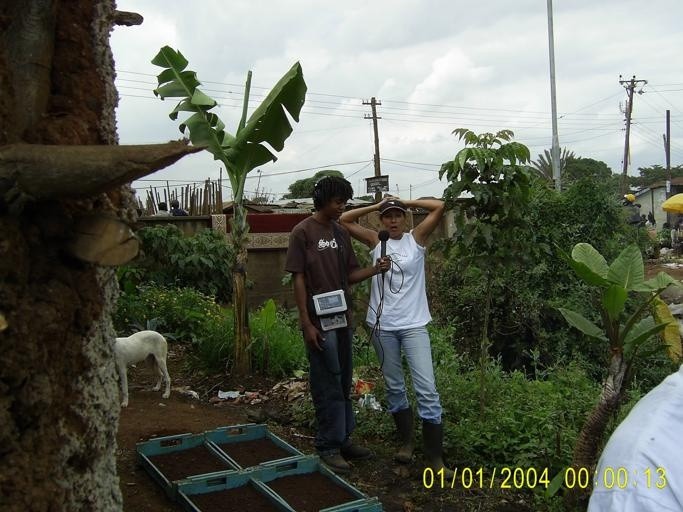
[420, 419, 456, 479]
[392, 404, 417, 464]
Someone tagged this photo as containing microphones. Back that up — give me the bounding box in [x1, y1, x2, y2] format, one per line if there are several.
[378, 231, 390, 273]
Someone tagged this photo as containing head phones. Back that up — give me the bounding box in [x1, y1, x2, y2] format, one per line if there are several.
[313, 176, 333, 204]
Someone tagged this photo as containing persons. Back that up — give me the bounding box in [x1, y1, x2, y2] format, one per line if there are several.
[586, 363, 683, 512]
[339, 194, 456, 480]
[283, 176, 391, 474]
[154, 200, 188, 216]
[622, 193, 683, 255]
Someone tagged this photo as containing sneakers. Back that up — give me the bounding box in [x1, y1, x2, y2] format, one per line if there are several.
[320, 450, 350, 474]
[340, 438, 372, 461]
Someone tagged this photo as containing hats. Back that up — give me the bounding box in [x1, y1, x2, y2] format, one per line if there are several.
[379, 200, 408, 215]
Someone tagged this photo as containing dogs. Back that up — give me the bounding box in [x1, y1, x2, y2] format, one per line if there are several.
[113, 330, 171, 408]
